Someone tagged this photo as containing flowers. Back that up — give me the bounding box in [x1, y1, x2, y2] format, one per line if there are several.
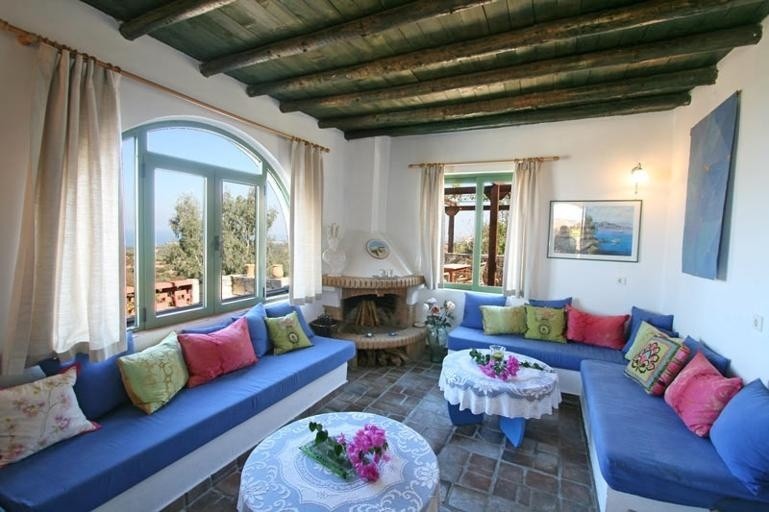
[422, 296, 454, 343]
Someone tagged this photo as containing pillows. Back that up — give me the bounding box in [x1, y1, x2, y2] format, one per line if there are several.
[73, 351, 129, 419]
[177, 316, 259, 387]
[264, 311, 314, 356]
[266, 304, 313, 340]
[623, 305, 768, 496]
[119, 330, 190, 414]
[0, 360, 103, 467]
[231, 302, 274, 357]
[461, 292, 630, 349]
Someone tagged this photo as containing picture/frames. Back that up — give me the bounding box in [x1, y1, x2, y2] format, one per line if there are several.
[546, 200, 642, 262]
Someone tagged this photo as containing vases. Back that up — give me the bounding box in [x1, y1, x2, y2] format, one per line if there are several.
[425, 316, 448, 363]
[272, 264, 283, 279]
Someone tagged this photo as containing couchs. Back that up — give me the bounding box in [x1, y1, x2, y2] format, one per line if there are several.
[445, 293, 769, 511]
[0, 337, 356, 512]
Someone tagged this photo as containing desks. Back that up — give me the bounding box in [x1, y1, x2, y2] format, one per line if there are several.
[444, 264, 471, 282]
[438, 348, 559, 447]
[238, 411, 441, 511]
